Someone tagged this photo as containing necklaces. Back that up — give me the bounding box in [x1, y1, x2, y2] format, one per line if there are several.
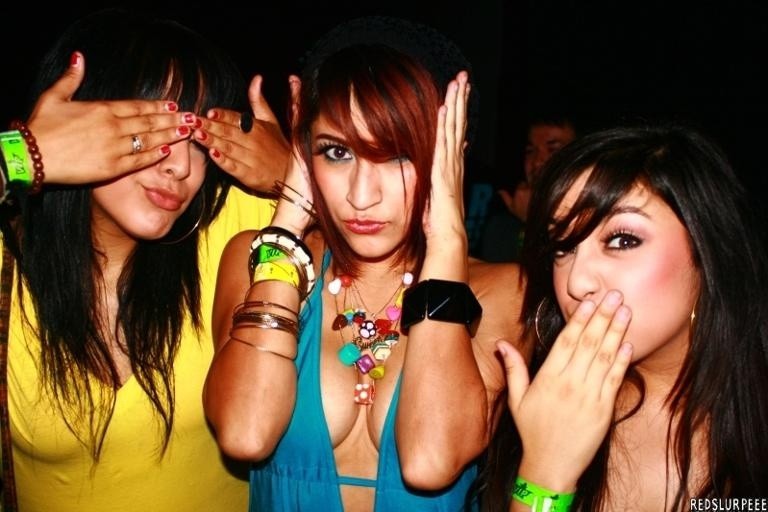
[327, 258, 415, 405]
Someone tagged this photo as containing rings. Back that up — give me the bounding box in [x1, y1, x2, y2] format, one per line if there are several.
[132, 135, 142, 153]
[239, 114, 254, 134]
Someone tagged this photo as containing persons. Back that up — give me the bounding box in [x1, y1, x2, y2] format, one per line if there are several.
[200, 34, 537, 512]
[465, 117, 768, 512]
[0, 12, 293, 512]
[500, 114, 582, 263]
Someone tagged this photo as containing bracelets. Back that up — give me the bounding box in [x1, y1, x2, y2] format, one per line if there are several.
[229, 177, 319, 362]
[400, 279, 482, 337]
[10, 118, 46, 194]
[1, 130, 33, 193]
[512, 475, 579, 512]
